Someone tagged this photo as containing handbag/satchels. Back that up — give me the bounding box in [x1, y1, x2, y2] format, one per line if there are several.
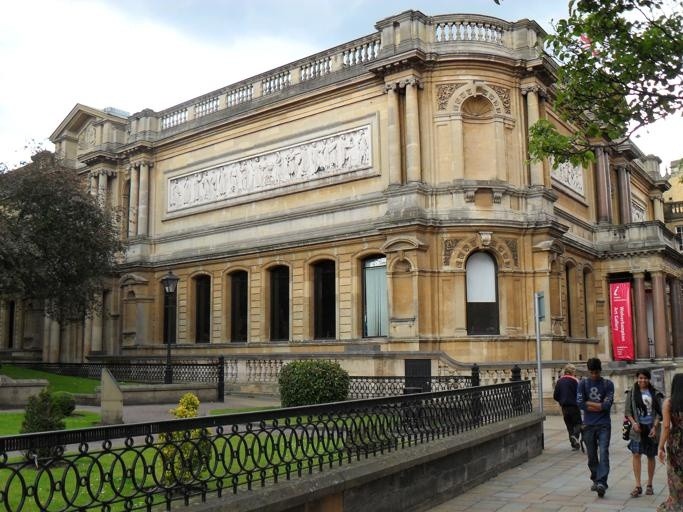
[621, 415, 632, 442]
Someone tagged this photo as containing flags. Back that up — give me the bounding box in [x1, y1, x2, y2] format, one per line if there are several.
[577, 32, 603, 56]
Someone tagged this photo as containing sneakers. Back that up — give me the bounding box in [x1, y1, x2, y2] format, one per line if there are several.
[570, 435, 580, 451]
[629, 486, 643, 498]
[597, 483, 605, 499]
[645, 484, 654, 496]
[590, 481, 598, 492]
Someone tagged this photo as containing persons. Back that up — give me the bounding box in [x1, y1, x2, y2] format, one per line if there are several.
[656, 373, 683, 512]
[553, 363, 580, 451]
[624, 369, 664, 497]
[577, 358, 614, 497]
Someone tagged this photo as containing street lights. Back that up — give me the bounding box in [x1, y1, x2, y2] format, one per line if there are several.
[159, 264, 187, 383]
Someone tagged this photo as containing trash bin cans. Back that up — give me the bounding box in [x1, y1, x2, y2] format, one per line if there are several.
[403, 387, 424, 428]
